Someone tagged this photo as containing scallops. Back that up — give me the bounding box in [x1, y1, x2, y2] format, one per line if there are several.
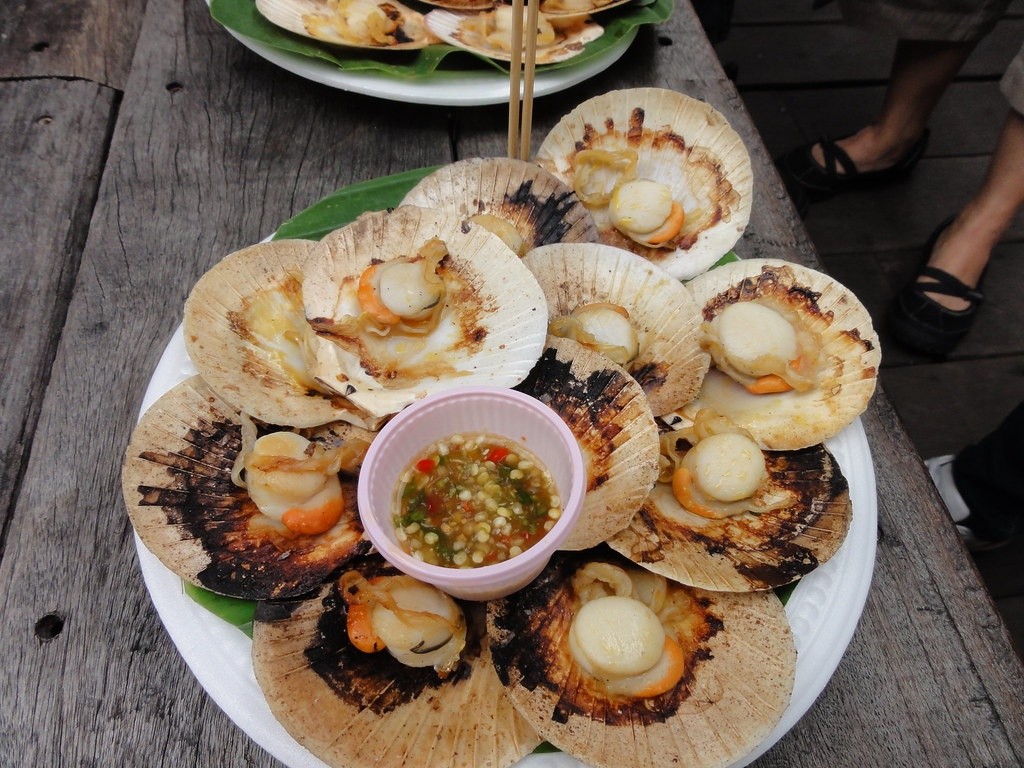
[120, 0, 882, 768]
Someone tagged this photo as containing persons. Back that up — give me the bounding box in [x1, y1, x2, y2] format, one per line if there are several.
[793, 1, 1023, 354]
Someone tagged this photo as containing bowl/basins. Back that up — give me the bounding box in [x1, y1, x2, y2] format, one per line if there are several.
[358, 387, 586, 601]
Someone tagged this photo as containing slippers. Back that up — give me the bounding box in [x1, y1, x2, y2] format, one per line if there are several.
[800, 123, 934, 191]
[887, 207, 999, 362]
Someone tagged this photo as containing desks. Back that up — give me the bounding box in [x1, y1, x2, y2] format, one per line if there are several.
[0, 0, 1024, 768]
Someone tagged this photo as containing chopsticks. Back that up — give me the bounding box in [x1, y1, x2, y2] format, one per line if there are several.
[507, 1, 538, 162]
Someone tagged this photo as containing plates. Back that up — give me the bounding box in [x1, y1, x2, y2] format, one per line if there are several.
[133, 228, 877, 768]
[206, 1, 639, 105]
